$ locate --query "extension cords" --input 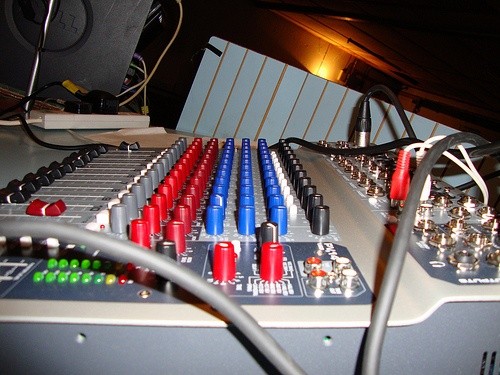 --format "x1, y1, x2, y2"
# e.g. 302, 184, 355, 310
28, 111, 150, 129
